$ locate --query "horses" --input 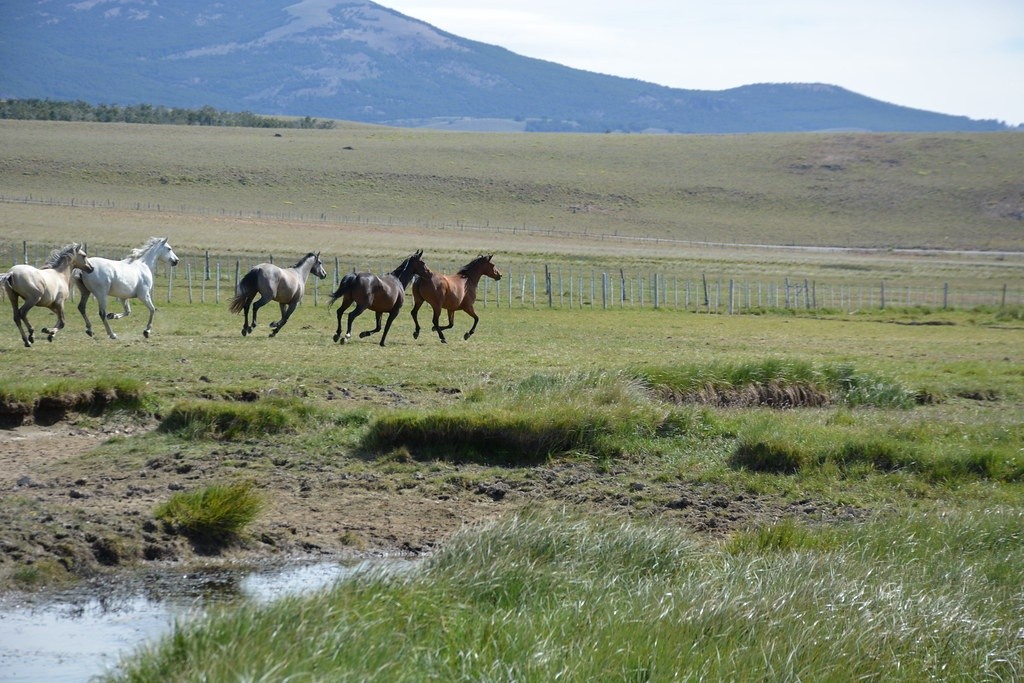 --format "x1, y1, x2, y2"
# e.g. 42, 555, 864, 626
326, 249, 433, 347
411, 253, 503, 344
226, 250, 327, 337
68, 235, 180, 339
0, 243, 94, 348
